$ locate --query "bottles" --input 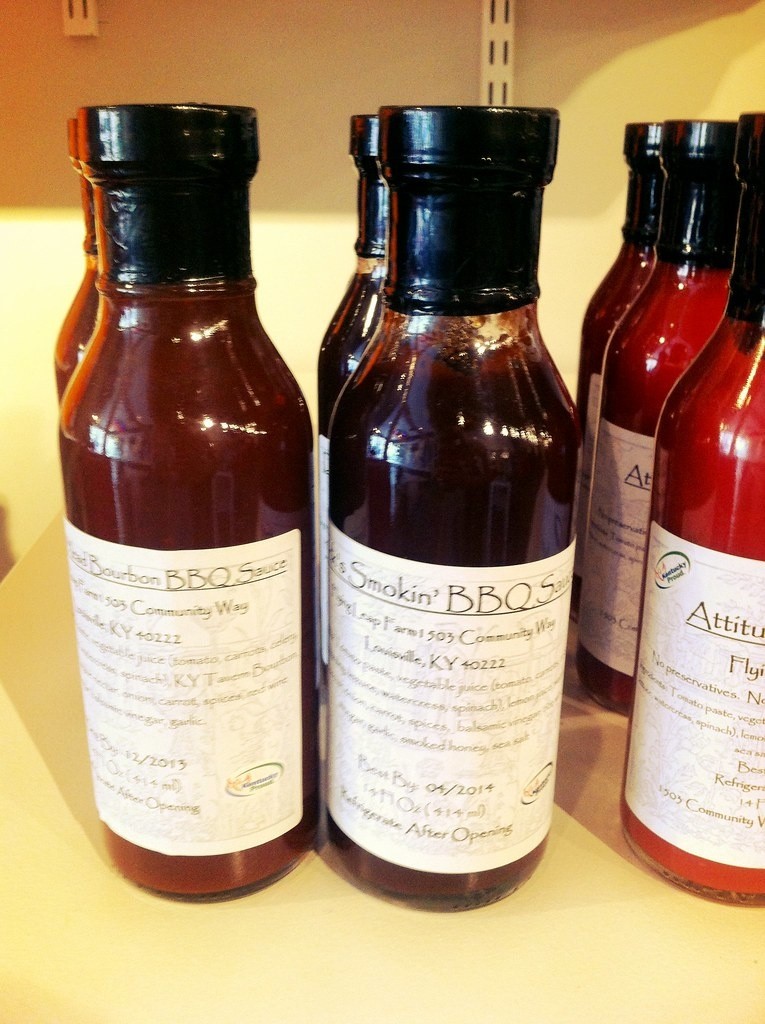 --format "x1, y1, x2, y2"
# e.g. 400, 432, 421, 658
328, 105, 582, 911
567, 123, 664, 625
318, 114, 389, 688
621, 112, 765, 908
54, 119, 101, 406
576, 119, 743, 714
58, 102, 313, 903
